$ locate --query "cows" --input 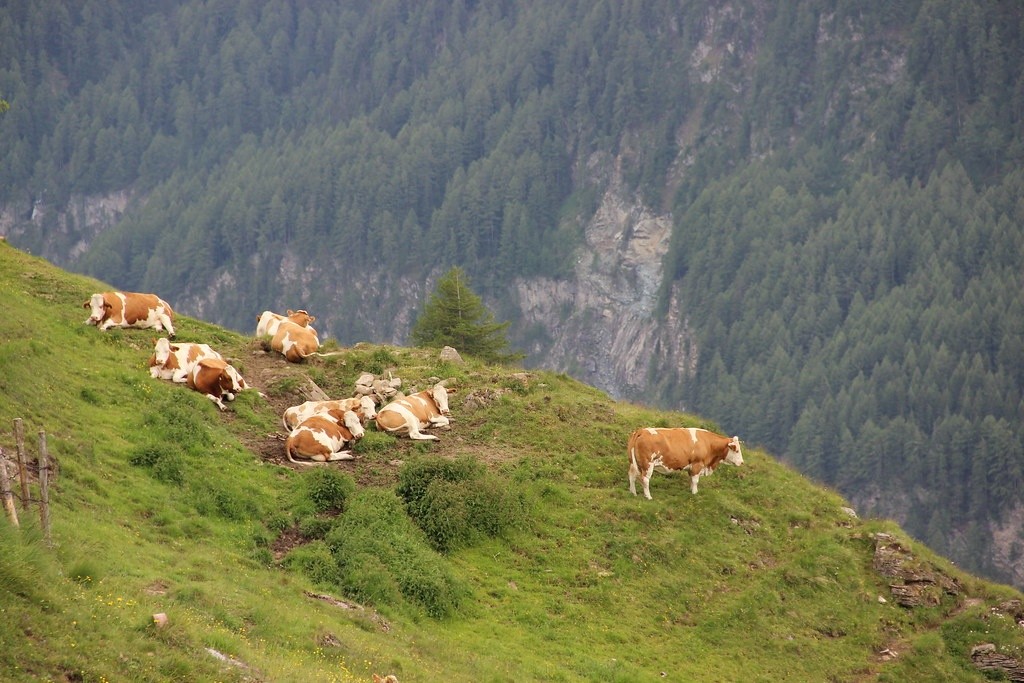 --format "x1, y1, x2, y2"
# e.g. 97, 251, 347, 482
376, 385, 457, 442
282, 396, 378, 466
148, 337, 267, 413
627, 427, 744, 500
82, 291, 176, 340
256, 309, 321, 363
